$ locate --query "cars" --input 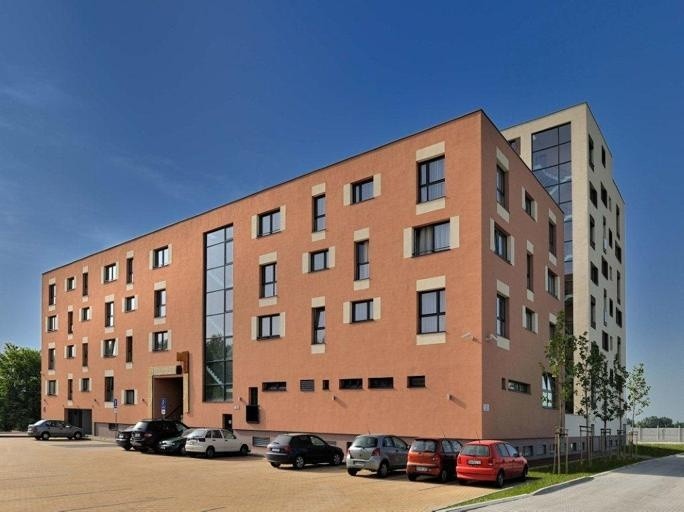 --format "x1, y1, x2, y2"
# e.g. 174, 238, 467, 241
262, 432, 346, 471
114, 418, 252, 459
456, 437, 531, 488
402, 436, 461, 483
343, 432, 412, 478
26, 418, 86, 442
